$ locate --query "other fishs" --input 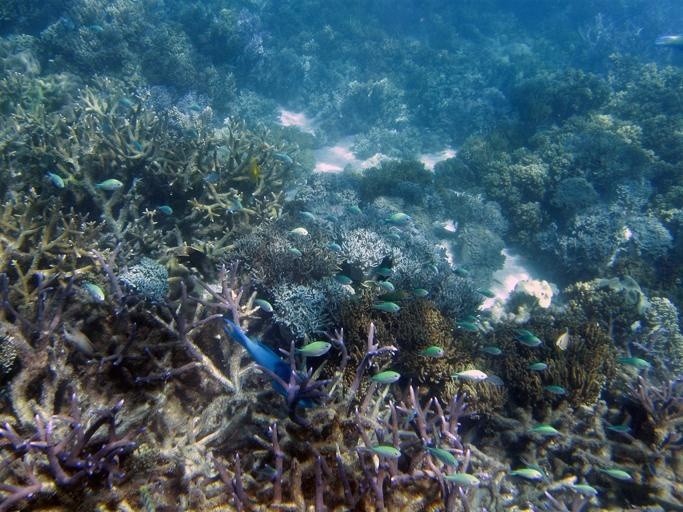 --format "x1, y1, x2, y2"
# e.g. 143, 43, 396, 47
332, 267, 650, 496
